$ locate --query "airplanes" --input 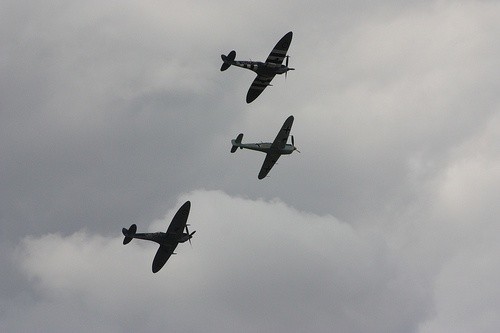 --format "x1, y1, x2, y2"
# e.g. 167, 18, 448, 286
122, 200, 197, 273
231, 115, 301, 180
220, 31, 295, 104
